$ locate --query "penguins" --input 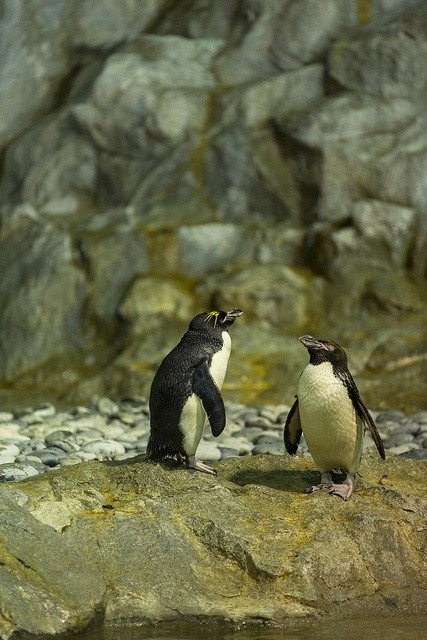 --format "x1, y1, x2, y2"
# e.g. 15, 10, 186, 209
284, 336, 385, 500
145, 309, 243, 477
